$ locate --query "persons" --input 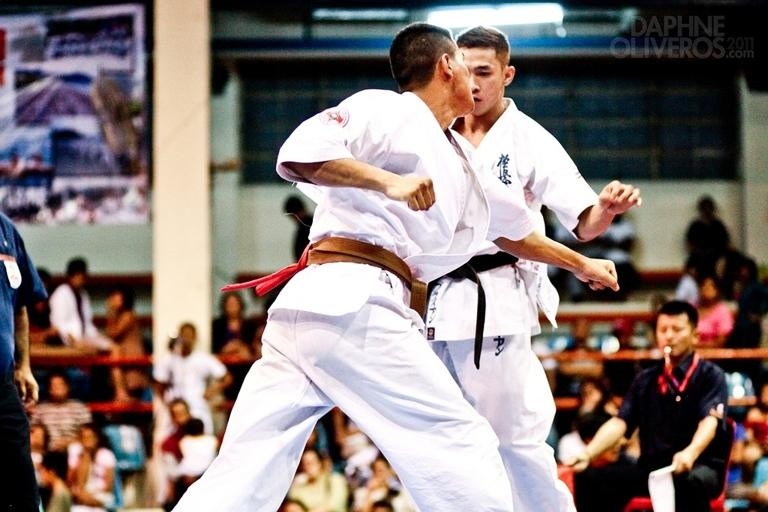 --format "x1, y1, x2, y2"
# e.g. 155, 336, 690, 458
15, 248, 768, 511
542, 200, 640, 301
446, 26, 642, 512
1, 211, 50, 510
684, 195, 731, 273
284, 196, 316, 263
172, 23, 622, 511
